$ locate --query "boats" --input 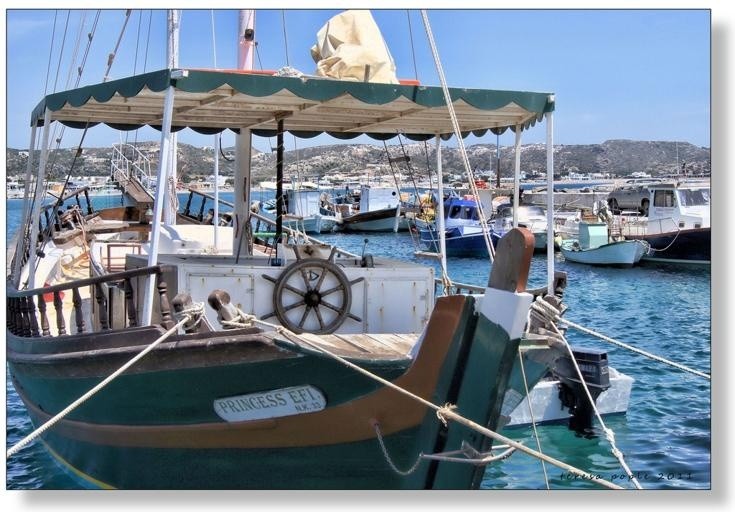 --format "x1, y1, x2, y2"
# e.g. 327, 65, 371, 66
7, 10, 710, 493
